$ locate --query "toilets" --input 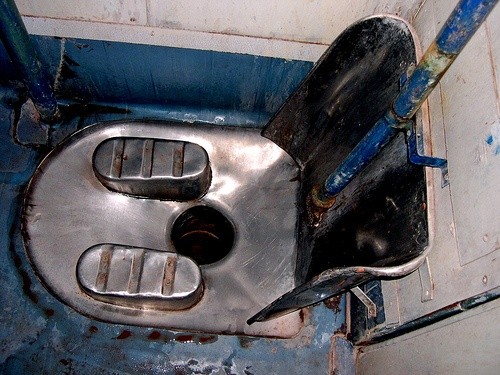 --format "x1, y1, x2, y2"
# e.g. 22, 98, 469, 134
23, 15, 435, 338
